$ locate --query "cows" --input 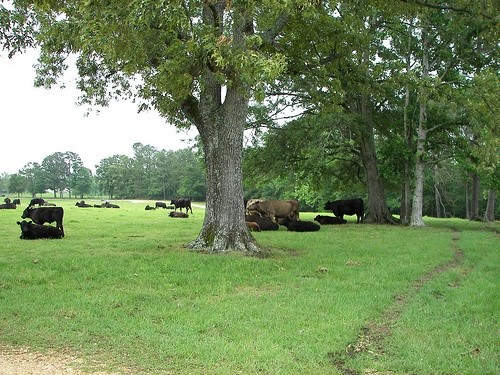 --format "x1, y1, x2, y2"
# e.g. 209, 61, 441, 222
22, 206, 65, 238
0, 198, 21, 210
244, 198, 300, 231
16, 221, 63, 239
326, 198, 365, 224
171, 199, 193, 214
314, 215, 347, 225
170, 211, 189, 218
29, 198, 56, 207
75, 201, 120, 209
145, 201, 174, 210
279, 217, 321, 232
